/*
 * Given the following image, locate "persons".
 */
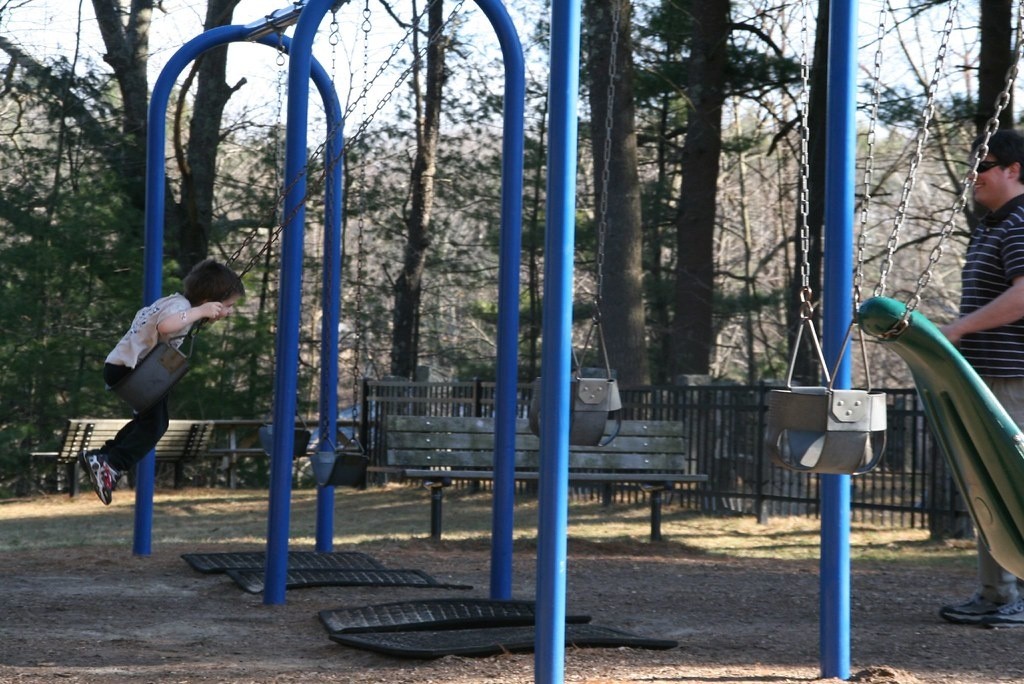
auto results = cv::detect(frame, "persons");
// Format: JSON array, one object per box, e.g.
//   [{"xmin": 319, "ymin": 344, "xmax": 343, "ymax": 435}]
[
  {"xmin": 936, "ymin": 130, "xmax": 1024, "ymax": 628},
  {"xmin": 78, "ymin": 259, "xmax": 245, "ymax": 506}
]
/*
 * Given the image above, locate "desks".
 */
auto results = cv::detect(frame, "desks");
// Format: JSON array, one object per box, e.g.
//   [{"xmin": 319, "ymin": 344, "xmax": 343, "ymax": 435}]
[{"xmin": 211, "ymin": 420, "xmax": 382, "ymax": 491}]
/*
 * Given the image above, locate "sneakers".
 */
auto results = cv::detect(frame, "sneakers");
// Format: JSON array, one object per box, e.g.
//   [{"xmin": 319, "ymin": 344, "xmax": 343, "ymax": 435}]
[
  {"xmin": 86, "ymin": 454, "xmax": 120, "ymax": 506},
  {"xmin": 939, "ymin": 595, "xmax": 1024, "ymax": 627},
  {"xmin": 79, "ymin": 450, "xmax": 92, "ymax": 479}
]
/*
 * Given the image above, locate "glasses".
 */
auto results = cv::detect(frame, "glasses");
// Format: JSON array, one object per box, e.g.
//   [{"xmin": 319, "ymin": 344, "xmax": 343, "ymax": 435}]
[{"xmin": 976, "ymin": 160, "xmax": 1000, "ymax": 174}]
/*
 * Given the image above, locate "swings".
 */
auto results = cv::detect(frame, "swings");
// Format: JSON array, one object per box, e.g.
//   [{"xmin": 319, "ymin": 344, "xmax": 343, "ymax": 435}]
[
  {"xmin": 514, "ymin": 1, "xmax": 626, "ymax": 452},
  {"xmin": 313, "ymin": 0, "xmax": 371, "ymax": 481},
  {"xmin": 133, "ymin": 0, "xmax": 466, "ymax": 402},
  {"xmin": 760, "ymin": 0, "xmax": 888, "ymax": 474},
  {"xmin": 259, "ymin": 23, "xmax": 312, "ymax": 459}
]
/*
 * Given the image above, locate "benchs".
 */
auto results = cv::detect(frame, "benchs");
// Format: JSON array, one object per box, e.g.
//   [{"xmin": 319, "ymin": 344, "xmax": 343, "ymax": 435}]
[
  {"xmin": 29, "ymin": 418, "xmax": 214, "ymax": 497},
  {"xmin": 200, "ymin": 447, "xmax": 361, "ymax": 455},
  {"xmin": 387, "ymin": 413, "xmax": 709, "ymax": 544}
]
